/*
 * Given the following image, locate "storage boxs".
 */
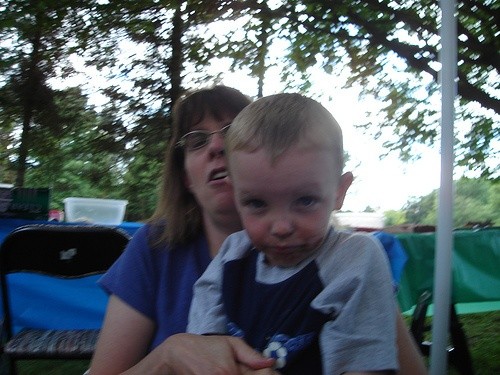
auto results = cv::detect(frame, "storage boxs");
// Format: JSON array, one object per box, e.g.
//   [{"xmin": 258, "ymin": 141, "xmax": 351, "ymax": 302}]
[{"xmin": 62, "ymin": 197, "xmax": 129, "ymax": 226}]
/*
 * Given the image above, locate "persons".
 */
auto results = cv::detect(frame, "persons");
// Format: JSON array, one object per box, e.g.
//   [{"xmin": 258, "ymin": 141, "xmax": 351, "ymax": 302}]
[
  {"xmin": 87, "ymin": 84, "xmax": 429, "ymax": 375},
  {"xmin": 185, "ymin": 92, "xmax": 400, "ymax": 375}
]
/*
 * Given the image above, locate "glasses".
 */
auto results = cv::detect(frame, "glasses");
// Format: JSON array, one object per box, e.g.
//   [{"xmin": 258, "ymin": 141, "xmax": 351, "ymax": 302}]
[{"xmin": 176, "ymin": 124, "xmax": 231, "ymax": 149}]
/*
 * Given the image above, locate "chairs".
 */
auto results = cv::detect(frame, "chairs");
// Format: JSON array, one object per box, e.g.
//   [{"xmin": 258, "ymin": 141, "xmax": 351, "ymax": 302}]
[{"xmin": 0, "ymin": 224, "xmax": 132, "ymax": 375}]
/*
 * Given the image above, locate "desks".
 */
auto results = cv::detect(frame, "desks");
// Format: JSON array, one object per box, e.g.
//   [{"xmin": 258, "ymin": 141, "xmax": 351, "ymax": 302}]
[{"xmin": 0, "ymin": 219, "xmax": 144, "ymax": 247}]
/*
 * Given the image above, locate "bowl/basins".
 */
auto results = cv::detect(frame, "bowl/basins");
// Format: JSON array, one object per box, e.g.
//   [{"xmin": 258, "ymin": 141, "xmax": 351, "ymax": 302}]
[
  {"xmin": 334, "ymin": 212, "xmax": 383, "ymax": 233},
  {"xmin": 62, "ymin": 197, "xmax": 128, "ymax": 225}
]
